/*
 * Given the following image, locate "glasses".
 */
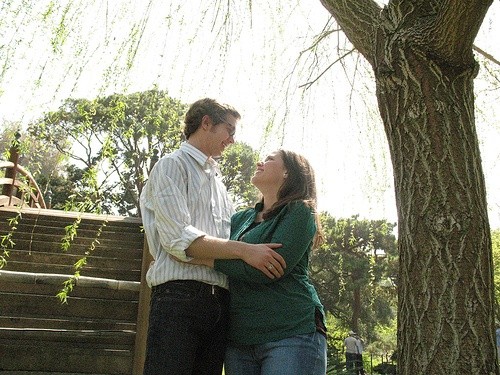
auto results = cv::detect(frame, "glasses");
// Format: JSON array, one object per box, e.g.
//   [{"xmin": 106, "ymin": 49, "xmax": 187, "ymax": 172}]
[{"xmin": 217, "ymin": 116, "xmax": 236, "ymax": 136}]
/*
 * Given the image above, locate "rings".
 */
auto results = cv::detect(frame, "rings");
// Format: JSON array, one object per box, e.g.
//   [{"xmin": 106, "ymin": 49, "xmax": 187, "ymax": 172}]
[{"xmin": 268, "ymin": 264, "xmax": 273, "ymax": 270}]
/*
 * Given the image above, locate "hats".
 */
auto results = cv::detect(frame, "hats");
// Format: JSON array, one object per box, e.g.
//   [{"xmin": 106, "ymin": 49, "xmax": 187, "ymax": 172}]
[{"xmin": 349, "ymin": 330, "xmax": 356, "ymax": 336}]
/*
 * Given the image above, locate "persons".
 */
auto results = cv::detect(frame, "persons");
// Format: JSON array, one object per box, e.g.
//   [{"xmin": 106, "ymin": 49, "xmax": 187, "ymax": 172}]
[
  {"xmin": 169, "ymin": 148, "xmax": 327, "ymax": 375},
  {"xmin": 343, "ymin": 331, "xmax": 359, "ymax": 373},
  {"xmin": 353, "ymin": 333, "xmax": 365, "ymax": 375},
  {"xmin": 139, "ymin": 98, "xmax": 287, "ymax": 375},
  {"xmin": 496, "ymin": 323, "xmax": 500, "ymax": 366}
]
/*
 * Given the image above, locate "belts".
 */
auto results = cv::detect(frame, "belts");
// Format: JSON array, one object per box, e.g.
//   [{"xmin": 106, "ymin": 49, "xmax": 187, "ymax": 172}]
[{"xmin": 151, "ymin": 279, "xmax": 231, "ymax": 302}]
